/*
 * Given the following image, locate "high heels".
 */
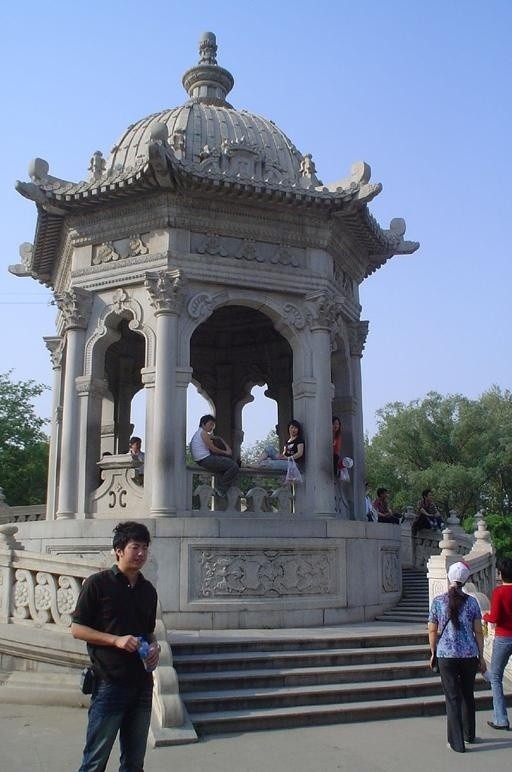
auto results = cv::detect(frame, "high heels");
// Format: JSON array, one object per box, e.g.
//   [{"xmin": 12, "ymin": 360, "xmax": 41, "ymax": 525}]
[{"xmin": 487, "ymin": 721, "xmax": 509, "ymax": 731}]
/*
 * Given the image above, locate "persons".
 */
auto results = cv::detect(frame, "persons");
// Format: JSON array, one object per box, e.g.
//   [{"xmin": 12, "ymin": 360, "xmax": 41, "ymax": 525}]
[
  {"xmin": 412, "ymin": 489, "xmax": 442, "ymax": 535},
  {"xmin": 427, "ymin": 560, "xmax": 489, "ymax": 752},
  {"xmin": 374, "ymin": 488, "xmax": 404, "ymax": 524},
  {"xmin": 239, "ymin": 420, "xmax": 304, "ymax": 472},
  {"xmin": 484, "ymin": 558, "xmax": 512, "ymax": 730},
  {"xmin": 71, "ymin": 520, "xmax": 163, "ymax": 772},
  {"xmin": 126, "ymin": 437, "xmax": 145, "ymax": 487},
  {"xmin": 331, "ymin": 416, "xmax": 341, "ymax": 477},
  {"xmin": 100, "ymin": 451, "xmax": 112, "ymax": 484},
  {"xmin": 190, "ymin": 415, "xmax": 238, "ymax": 496},
  {"xmin": 366, "ymin": 482, "xmax": 373, "ymax": 522}
]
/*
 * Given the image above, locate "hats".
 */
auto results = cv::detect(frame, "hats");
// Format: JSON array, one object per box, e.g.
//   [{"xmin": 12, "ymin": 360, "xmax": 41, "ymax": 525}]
[{"xmin": 447, "ymin": 562, "xmax": 470, "ymax": 584}]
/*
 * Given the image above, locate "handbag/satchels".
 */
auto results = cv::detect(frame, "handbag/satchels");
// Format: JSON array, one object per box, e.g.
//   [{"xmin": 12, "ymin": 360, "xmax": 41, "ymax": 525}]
[{"xmin": 80, "ymin": 666, "xmax": 96, "ymax": 694}]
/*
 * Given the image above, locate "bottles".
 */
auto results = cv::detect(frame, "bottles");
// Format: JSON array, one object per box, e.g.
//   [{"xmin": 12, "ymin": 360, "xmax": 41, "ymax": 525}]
[
  {"xmin": 481, "ymin": 619, "xmax": 488, "ymax": 639},
  {"xmin": 135, "ymin": 635, "xmax": 155, "ymax": 672},
  {"xmin": 478, "ymin": 664, "xmax": 492, "ymax": 682}
]
